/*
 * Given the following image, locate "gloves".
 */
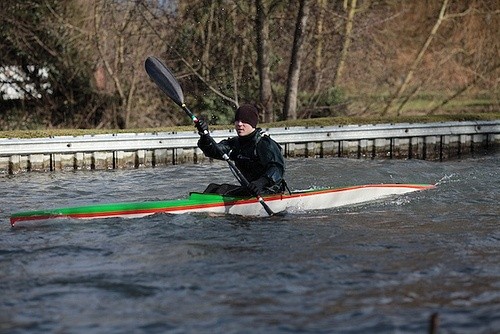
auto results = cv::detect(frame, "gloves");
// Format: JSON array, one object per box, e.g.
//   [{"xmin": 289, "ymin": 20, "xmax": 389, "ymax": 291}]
[
  {"xmin": 247, "ymin": 176, "xmax": 271, "ymax": 197},
  {"xmin": 194, "ymin": 120, "xmax": 209, "ymax": 136}
]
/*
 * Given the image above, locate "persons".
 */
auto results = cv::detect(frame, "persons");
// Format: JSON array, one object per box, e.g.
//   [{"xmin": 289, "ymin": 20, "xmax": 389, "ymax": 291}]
[{"xmin": 196, "ymin": 104, "xmax": 286, "ymax": 196}]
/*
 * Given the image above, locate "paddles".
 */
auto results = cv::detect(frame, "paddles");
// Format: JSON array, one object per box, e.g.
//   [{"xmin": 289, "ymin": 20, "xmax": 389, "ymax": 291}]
[{"xmin": 143, "ymin": 55, "xmax": 284, "ymax": 218}]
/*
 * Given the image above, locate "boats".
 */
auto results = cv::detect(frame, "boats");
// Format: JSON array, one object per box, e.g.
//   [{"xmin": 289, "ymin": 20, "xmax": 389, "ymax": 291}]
[{"xmin": 10, "ymin": 183, "xmax": 438, "ymax": 227}]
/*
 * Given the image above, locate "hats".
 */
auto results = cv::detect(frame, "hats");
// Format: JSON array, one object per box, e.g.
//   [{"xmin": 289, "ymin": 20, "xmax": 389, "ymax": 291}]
[{"xmin": 234, "ymin": 104, "xmax": 259, "ymax": 128}]
